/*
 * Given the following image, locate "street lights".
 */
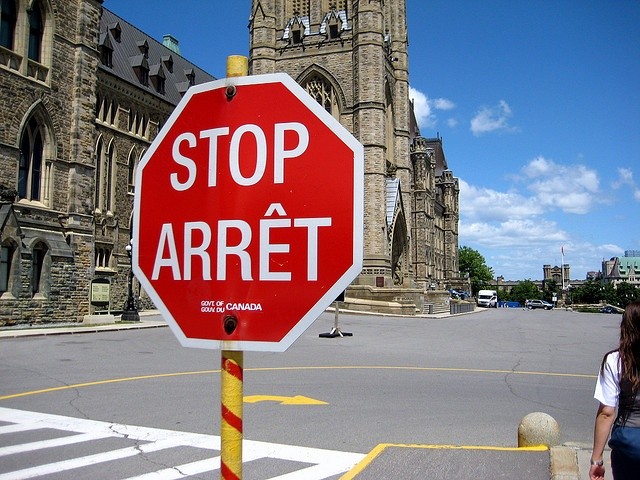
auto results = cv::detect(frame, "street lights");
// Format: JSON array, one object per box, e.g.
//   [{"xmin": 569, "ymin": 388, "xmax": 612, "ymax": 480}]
[
  {"xmin": 600, "ymin": 286, "xmax": 605, "ymax": 301},
  {"xmin": 496, "ymin": 274, "xmax": 505, "ymax": 303},
  {"xmin": 626, "ymin": 293, "xmax": 634, "ymax": 303}
]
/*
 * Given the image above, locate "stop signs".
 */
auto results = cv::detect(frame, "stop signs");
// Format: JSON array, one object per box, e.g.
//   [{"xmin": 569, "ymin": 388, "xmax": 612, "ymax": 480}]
[{"xmin": 130, "ymin": 72, "xmax": 363, "ymax": 349}]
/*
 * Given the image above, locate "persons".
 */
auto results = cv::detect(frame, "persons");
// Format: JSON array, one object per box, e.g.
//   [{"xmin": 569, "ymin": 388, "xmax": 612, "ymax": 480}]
[{"xmin": 588, "ymin": 304, "xmax": 640, "ymax": 480}]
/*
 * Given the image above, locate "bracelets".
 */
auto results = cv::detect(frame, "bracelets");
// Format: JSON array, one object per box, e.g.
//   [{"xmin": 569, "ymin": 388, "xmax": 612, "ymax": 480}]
[{"xmin": 590, "ymin": 460, "xmax": 605, "ymax": 466}]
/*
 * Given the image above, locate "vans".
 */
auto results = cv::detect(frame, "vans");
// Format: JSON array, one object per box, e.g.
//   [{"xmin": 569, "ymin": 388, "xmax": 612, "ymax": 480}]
[{"xmin": 478, "ymin": 290, "xmax": 497, "ymax": 308}]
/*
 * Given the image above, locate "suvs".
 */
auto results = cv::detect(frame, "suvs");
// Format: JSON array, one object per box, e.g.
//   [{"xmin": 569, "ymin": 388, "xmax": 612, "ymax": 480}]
[{"xmin": 526, "ymin": 299, "xmax": 554, "ymax": 310}]
